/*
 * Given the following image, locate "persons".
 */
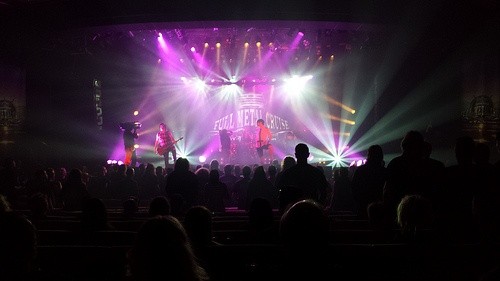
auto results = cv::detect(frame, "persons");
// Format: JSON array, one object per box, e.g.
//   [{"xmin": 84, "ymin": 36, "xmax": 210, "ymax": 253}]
[
  {"xmin": 310, "ymin": 130, "xmax": 500, "ymax": 251},
  {"xmin": 0, "ymin": 211, "xmax": 43, "ymax": 281},
  {"xmin": 257, "ymin": 200, "xmax": 357, "ymax": 281},
  {"xmin": 256, "ymin": 119, "xmax": 275, "ymax": 166},
  {"xmin": 219, "ymin": 130, "xmax": 233, "ymax": 166},
  {"xmin": 285, "ymin": 131, "xmax": 298, "ymax": 158},
  {"xmin": 123, "ymin": 124, "xmax": 139, "ymax": 170},
  {"xmin": 155, "ymin": 122, "xmax": 177, "ymax": 168},
  {"xmin": 0, "ymin": 143, "xmax": 311, "ymax": 244},
  {"xmin": 126, "ymin": 216, "xmax": 213, "ymax": 281}
]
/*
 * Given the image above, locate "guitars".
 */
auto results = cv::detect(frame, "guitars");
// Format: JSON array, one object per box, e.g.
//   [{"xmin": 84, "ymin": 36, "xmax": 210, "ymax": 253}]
[{"xmin": 157, "ymin": 137, "xmax": 183, "ymax": 155}]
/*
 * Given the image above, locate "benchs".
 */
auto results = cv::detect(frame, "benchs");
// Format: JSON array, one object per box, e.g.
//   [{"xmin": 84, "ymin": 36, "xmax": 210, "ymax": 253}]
[{"xmin": 36, "ymin": 209, "xmax": 402, "ymax": 281}]
